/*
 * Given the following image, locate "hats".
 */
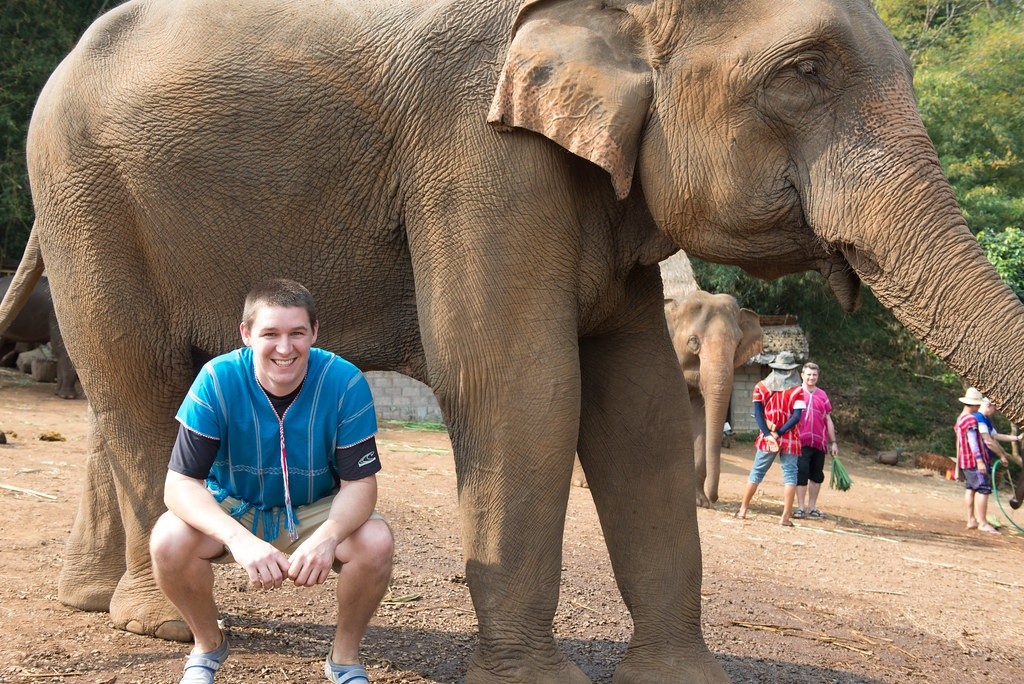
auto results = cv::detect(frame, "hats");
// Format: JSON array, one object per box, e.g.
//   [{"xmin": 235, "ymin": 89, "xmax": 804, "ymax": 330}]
[
  {"xmin": 765, "ymin": 351, "xmax": 800, "ymax": 391},
  {"xmin": 959, "ymin": 387, "xmax": 984, "ymax": 405}
]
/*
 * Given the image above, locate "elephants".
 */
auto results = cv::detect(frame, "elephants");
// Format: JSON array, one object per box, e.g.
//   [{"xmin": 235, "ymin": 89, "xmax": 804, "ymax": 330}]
[{"xmin": 1, "ymin": 0, "xmax": 1024, "ymax": 684}]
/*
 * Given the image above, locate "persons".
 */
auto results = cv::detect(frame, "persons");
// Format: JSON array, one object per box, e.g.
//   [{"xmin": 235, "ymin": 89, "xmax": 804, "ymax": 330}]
[
  {"xmin": 973, "ymin": 397, "xmax": 1023, "ymax": 529},
  {"xmin": 148, "ymin": 277, "xmax": 394, "ymax": 684},
  {"xmin": 953, "ymin": 387, "xmax": 1001, "ymax": 536},
  {"xmin": 736, "ymin": 351, "xmax": 807, "ymax": 527},
  {"xmin": 792, "ymin": 362, "xmax": 839, "ymax": 519}
]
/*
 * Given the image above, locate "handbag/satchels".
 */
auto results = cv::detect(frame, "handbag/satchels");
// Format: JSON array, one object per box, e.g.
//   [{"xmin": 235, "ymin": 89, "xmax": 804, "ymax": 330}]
[{"xmin": 954, "ymin": 454, "xmax": 965, "ymax": 482}]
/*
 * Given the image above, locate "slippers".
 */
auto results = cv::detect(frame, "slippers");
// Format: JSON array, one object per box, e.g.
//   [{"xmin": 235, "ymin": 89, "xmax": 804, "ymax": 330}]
[
  {"xmin": 809, "ymin": 510, "xmax": 824, "ymax": 518},
  {"xmin": 735, "ymin": 510, "xmax": 745, "ymax": 520},
  {"xmin": 792, "ymin": 509, "xmax": 806, "ymax": 519},
  {"xmin": 782, "ymin": 521, "xmax": 795, "ymax": 527}
]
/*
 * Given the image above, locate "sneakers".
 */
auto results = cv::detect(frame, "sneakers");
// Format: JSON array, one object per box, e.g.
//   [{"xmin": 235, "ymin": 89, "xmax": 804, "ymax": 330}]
[
  {"xmin": 323, "ymin": 645, "xmax": 369, "ymax": 684},
  {"xmin": 178, "ymin": 629, "xmax": 230, "ymax": 684}
]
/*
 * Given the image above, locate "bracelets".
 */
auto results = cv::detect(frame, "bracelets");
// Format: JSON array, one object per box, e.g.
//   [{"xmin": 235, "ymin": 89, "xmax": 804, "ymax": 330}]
[
  {"xmin": 831, "ymin": 441, "xmax": 836, "ymax": 444},
  {"xmin": 975, "ymin": 455, "xmax": 982, "ymax": 459},
  {"xmin": 1016, "ymin": 436, "xmax": 1019, "ymax": 442}
]
